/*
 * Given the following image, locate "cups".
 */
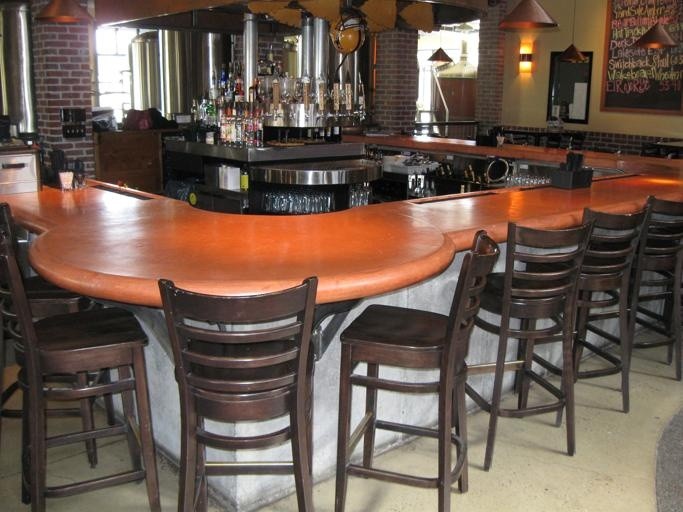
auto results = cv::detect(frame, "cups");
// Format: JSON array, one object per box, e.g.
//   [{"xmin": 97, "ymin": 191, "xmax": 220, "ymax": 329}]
[
  {"xmin": 58, "ymin": 171, "xmax": 73, "ymax": 190},
  {"xmin": 263, "ymin": 184, "xmax": 370, "ymax": 215}
]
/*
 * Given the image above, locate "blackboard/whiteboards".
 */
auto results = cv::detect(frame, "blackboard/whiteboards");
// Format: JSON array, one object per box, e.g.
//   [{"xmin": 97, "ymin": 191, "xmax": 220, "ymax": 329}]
[{"xmin": 600, "ymin": 0, "xmax": 683, "ymax": 117}]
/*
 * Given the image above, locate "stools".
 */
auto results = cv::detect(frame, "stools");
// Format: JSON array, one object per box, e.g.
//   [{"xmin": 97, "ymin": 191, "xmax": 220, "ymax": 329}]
[
  {"xmin": 1, "ymin": 203, "xmax": 162, "ymax": 512},
  {"xmin": 335, "ymin": 231, "xmax": 502, "ymax": 511},
  {"xmin": 158, "ymin": 276, "xmax": 316, "ymax": 512},
  {"xmin": 465, "ymin": 195, "xmax": 683, "ymax": 471}
]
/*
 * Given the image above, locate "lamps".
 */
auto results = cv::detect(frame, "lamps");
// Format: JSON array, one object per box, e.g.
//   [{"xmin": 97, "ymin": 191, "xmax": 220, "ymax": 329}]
[
  {"xmin": 425, "ymin": 30, "xmax": 454, "ymax": 63},
  {"xmin": 498, "ymin": 1, "xmax": 590, "ymax": 62},
  {"xmin": 633, "ymin": 1, "xmax": 679, "ymax": 50},
  {"xmin": 34, "ymin": 0, "xmax": 94, "ymax": 24}
]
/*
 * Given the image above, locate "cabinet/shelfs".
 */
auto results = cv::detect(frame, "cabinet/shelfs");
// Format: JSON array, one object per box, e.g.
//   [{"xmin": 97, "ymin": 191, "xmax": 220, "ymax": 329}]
[{"xmin": 93, "ymin": 125, "xmax": 190, "ymax": 195}]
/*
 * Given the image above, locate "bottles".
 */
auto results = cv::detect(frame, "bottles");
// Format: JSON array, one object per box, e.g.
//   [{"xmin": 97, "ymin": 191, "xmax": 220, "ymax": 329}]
[
  {"xmin": 506, "ymin": 173, "xmax": 552, "ymax": 188},
  {"xmin": 409, "ymin": 178, "xmax": 436, "ymax": 199},
  {"xmin": 190, "ymin": 60, "xmax": 341, "ymax": 149}
]
